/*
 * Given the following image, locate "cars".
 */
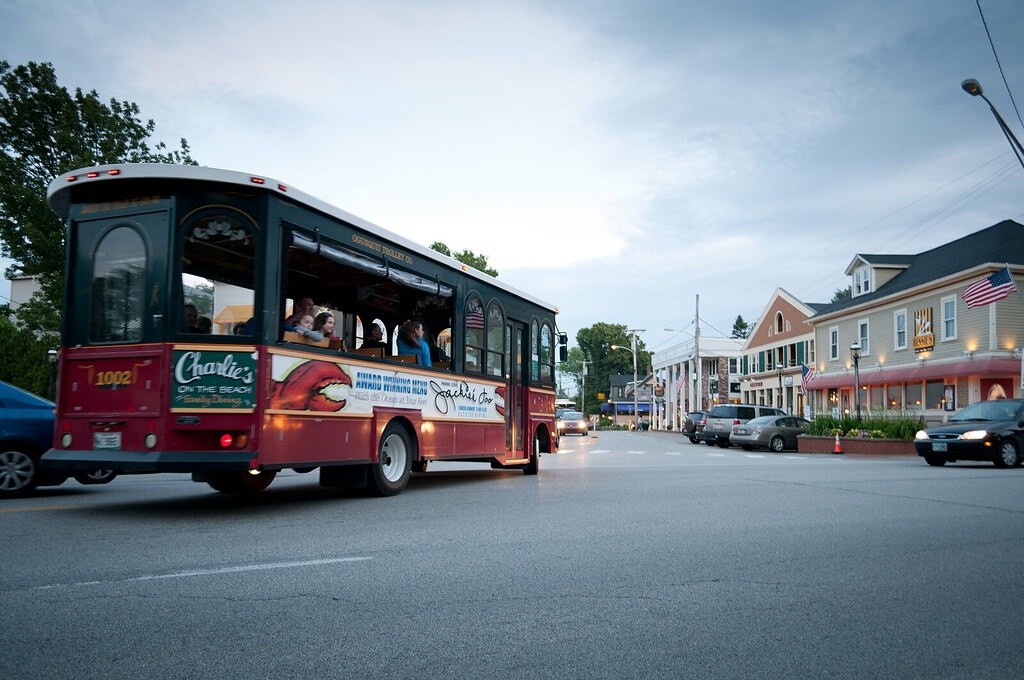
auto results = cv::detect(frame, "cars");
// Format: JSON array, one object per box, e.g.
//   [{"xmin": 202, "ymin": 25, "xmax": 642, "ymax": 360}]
[
  {"xmin": 729, "ymin": 415, "xmax": 816, "ymax": 453},
  {"xmin": 631, "ymin": 419, "xmax": 658, "ymax": 432},
  {"xmin": 556, "ymin": 399, "xmax": 588, "ymax": 436},
  {"xmin": 1, "ymin": 381, "xmax": 117, "ymax": 499},
  {"xmin": 913, "ymin": 399, "xmax": 1024, "ymax": 469}
]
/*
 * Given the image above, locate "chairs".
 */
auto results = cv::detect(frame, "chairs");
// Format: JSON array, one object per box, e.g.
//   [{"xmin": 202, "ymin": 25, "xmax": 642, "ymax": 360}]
[{"xmin": 284, "ymin": 331, "xmax": 493, "ymax": 376}]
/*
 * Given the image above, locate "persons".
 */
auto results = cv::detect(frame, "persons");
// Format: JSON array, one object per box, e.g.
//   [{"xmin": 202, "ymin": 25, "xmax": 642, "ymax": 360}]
[
  {"xmin": 360, "ymin": 323, "xmax": 390, "ymax": 356},
  {"xmin": 285, "ymin": 295, "xmax": 341, "ymax": 342},
  {"xmin": 396, "ymin": 320, "xmax": 432, "ymax": 368},
  {"xmin": 178, "ymin": 304, "xmax": 212, "ymax": 333},
  {"xmin": 423, "ymin": 333, "xmax": 446, "ymax": 362},
  {"xmin": 638, "ymin": 414, "xmax": 644, "ymax": 432}
]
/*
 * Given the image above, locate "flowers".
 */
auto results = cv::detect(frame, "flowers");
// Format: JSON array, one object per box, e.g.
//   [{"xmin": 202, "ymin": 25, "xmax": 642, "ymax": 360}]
[{"xmin": 830, "ymin": 429, "xmax": 886, "ymax": 438}]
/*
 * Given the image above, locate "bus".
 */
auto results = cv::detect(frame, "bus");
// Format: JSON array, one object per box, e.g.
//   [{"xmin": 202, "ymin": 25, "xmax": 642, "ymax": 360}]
[{"xmin": 40, "ymin": 163, "xmax": 568, "ymax": 498}]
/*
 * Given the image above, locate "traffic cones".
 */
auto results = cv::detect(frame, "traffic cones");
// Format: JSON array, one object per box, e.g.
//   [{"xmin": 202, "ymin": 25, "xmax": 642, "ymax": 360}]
[{"xmin": 831, "ymin": 432, "xmax": 844, "ymax": 454}]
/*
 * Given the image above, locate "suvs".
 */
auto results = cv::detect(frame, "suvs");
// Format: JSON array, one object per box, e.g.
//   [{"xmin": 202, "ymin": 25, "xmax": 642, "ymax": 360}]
[{"xmin": 682, "ymin": 404, "xmax": 788, "ymax": 448}]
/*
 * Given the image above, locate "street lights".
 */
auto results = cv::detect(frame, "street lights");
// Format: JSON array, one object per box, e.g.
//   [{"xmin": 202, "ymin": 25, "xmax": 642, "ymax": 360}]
[
  {"xmin": 664, "ymin": 327, "xmax": 699, "ymax": 413},
  {"xmin": 776, "ymin": 362, "xmax": 783, "ymax": 408},
  {"xmin": 611, "ymin": 345, "xmax": 638, "ymax": 432},
  {"xmin": 850, "ymin": 340, "xmax": 862, "ymax": 424}
]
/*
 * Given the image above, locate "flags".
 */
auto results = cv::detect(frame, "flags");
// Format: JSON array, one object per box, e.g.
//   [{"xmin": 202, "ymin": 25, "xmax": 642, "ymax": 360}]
[
  {"xmin": 801, "ymin": 364, "xmax": 814, "ymax": 395},
  {"xmin": 675, "ymin": 372, "xmax": 685, "ymax": 393},
  {"xmin": 961, "ymin": 267, "xmax": 1017, "ymax": 309}
]
